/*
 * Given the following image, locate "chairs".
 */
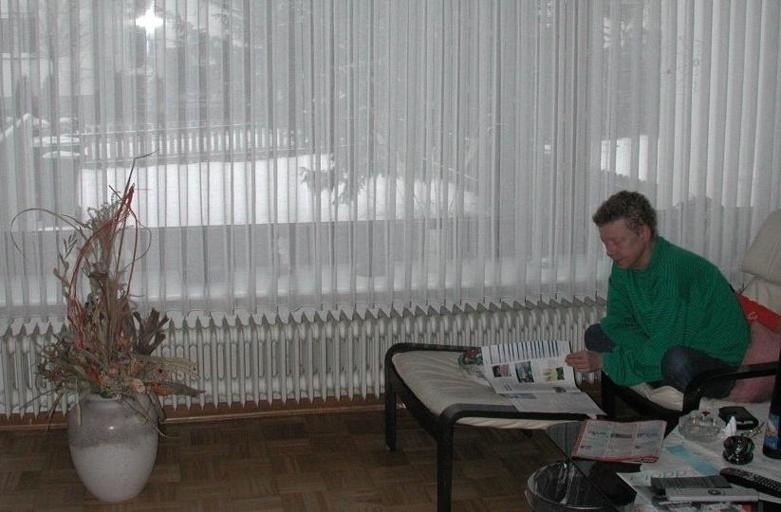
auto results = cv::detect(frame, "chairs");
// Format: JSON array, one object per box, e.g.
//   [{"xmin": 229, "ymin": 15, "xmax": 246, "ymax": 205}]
[{"xmin": 601, "ymin": 209, "xmax": 781, "ymax": 436}]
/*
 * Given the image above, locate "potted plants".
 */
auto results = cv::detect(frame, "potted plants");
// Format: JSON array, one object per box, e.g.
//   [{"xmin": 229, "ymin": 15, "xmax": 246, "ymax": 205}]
[{"xmin": 9, "ymin": 150, "xmax": 206, "ymax": 504}]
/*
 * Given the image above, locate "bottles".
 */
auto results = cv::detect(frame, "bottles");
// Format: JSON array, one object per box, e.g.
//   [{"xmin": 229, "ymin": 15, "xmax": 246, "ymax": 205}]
[{"xmin": 761, "ymin": 348, "xmax": 781, "ymax": 460}]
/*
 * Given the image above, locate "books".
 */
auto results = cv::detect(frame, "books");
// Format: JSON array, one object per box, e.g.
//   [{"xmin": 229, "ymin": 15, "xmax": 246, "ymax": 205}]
[
  {"xmin": 473, "ymin": 339, "xmax": 606, "ymax": 416},
  {"xmin": 572, "ymin": 418, "xmax": 672, "ymax": 464},
  {"xmin": 654, "ymin": 473, "xmax": 762, "ymax": 503}
]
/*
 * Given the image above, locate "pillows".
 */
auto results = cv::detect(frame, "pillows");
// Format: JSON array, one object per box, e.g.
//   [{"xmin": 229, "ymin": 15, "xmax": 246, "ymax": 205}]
[{"xmin": 724, "ymin": 321, "xmax": 781, "ymax": 403}]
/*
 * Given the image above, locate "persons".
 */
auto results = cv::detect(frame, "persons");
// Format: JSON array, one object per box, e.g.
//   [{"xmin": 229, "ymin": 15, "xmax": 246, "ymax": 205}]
[{"xmin": 564, "ymin": 188, "xmax": 749, "ymax": 397}]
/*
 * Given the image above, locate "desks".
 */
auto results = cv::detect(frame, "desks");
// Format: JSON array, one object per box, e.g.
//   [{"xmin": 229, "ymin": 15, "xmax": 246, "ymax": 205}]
[
  {"xmin": 545, "ymin": 412, "xmax": 781, "ymax": 512},
  {"xmin": 384, "ymin": 343, "xmax": 610, "ymax": 512}
]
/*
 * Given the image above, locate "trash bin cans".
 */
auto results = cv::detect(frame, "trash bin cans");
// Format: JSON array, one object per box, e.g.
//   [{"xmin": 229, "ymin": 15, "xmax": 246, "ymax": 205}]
[{"xmin": 525, "ymin": 462, "xmax": 606, "ymax": 511}]
